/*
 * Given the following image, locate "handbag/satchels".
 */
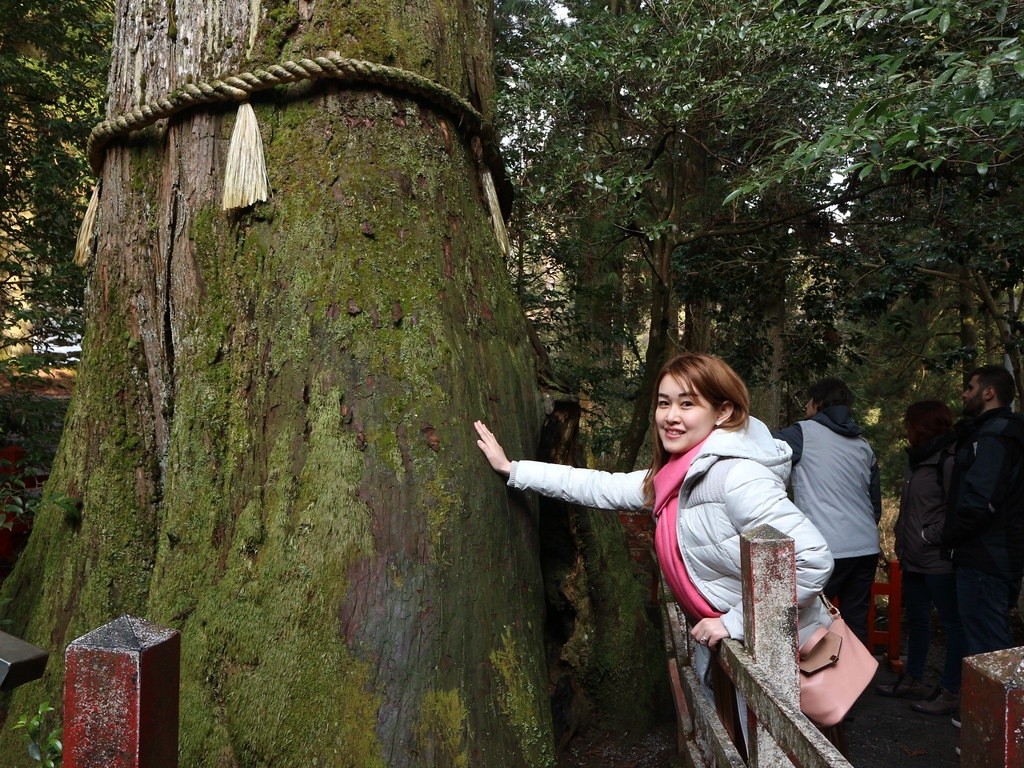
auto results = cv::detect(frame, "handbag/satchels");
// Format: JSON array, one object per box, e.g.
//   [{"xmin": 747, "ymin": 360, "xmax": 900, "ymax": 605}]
[{"xmin": 799, "ymin": 613, "xmax": 880, "ymax": 728}]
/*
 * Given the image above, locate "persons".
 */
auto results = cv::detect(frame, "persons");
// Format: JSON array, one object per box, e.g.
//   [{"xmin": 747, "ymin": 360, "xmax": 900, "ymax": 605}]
[
  {"xmin": 876, "ymin": 364, "xmax": 1024, "ymax": 755},
  {"xmin": 474, "ymin": 352, "xmax": 835, "ymax": 768},
  {"xmin": 773, "ymin": 376, "xmax": 882, "ymax": 644}
]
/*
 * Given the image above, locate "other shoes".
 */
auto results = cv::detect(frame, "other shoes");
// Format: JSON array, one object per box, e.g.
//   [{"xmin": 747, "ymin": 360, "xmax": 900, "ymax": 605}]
[{"xmin": 879, "ymin": 671, "xmax": 927, "ymax": 698}]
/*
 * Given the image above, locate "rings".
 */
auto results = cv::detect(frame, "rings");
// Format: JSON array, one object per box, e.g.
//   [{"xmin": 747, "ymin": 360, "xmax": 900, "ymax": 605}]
[{"xmin": 700, "ymin": 637, "xmax": 708, "ymax": 643}]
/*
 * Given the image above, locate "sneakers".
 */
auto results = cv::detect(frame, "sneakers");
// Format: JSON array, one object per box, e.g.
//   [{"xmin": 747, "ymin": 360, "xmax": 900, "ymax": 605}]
[{"xmin": 910, "ymin": 689, "xmax": 958, "ymax": 713}]
